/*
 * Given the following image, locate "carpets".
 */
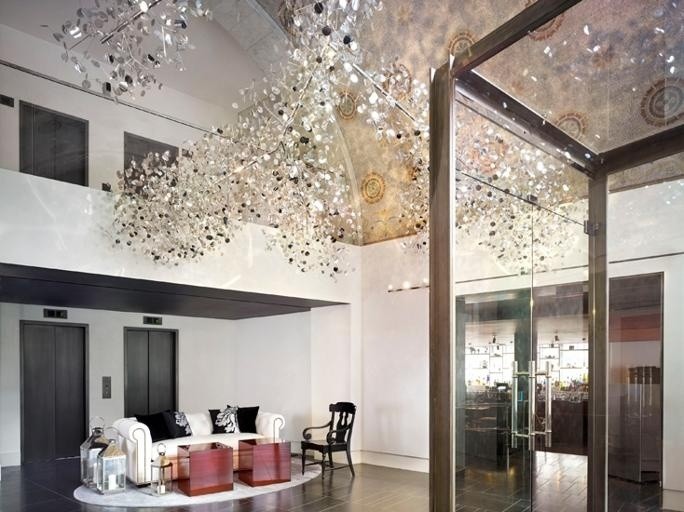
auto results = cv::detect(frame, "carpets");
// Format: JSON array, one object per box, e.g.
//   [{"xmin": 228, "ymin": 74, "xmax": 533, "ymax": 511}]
[{"xmin": 71, "ymin": 457, "xmax": 321, "ymax": 507}]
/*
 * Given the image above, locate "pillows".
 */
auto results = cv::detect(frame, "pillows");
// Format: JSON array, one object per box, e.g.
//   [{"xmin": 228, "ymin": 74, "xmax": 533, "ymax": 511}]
[{"xmin": 133, "ymin": 404, "xmax": 260, "ymax": 444}]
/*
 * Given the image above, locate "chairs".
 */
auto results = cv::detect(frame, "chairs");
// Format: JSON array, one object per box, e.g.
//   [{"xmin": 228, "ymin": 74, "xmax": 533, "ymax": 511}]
[{"xmin": 300, "ymin": 401, "xmax": 356, "ymax": 480}]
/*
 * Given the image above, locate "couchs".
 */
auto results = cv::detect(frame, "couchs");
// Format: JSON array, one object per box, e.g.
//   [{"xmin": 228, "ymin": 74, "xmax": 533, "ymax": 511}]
[{"xmin": 111, "ymin": 409, "xmax": 285, "ymax": 488}]
